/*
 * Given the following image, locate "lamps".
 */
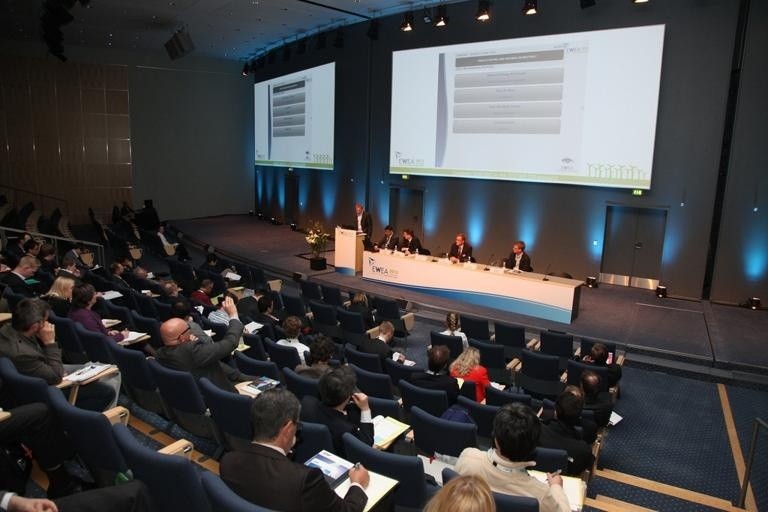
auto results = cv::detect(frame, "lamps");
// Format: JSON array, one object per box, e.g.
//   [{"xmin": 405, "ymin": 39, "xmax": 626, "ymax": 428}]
[
  {"xmin": 400, "ymin": 0, "xmax": 540, "ymax": 32},
  {"xmin": 241, "ymin": 28, "xmax": 344, "ymax": 75},
  {"xmin": 635, "ymin": 241, "xmax": 643, "ymax": 248}
]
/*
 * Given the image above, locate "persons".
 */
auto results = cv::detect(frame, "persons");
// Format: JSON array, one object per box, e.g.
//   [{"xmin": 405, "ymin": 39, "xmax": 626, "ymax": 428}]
[{"xmin": 1, "ymin": 201, "xmax": 622, "ymax": 511}]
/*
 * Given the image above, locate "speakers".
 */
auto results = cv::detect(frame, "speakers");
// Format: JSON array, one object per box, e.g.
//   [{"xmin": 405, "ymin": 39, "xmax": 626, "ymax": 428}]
[{"xmin": 164, "ymin": 28, "xmax": 196, "ymax": 61}]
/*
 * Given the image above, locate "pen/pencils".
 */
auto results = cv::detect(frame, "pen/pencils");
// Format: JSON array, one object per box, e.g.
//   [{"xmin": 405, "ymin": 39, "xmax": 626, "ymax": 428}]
[
  {"xmin": 354, "ymin": 462, "xmax": 360, "ymax": 472},
  {"xmin": 77, "ymin": 365, "xmax": 96, "ymax": 375},
  {"xmin": 545, "ymin": 468, "xmax": 562, "ymax": 482}
]
